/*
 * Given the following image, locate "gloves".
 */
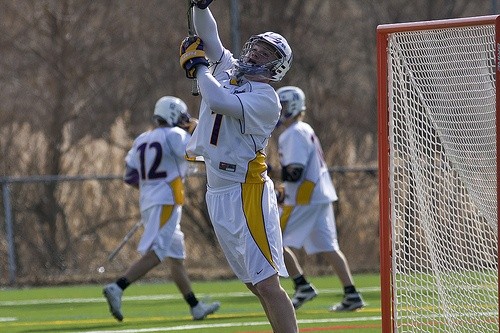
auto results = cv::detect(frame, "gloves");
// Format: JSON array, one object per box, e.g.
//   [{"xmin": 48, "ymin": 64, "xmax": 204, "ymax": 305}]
[
  {"xmin": 179, "ymin": 35, "xmax": 211, "ymax": 81},
  {"xmin": 192, "ymin": 0, "xmax": 214, "ymax": 12}
]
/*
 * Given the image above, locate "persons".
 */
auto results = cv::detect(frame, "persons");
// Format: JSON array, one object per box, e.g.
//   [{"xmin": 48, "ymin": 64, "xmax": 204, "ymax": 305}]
[
  {"xmin": 180, "ymin": 0, "xmax": 300, "ymax": 333},
  {"xmin": 275, "ymin": 86, "xmax": 367, "ymax": 312},
  {"xmin": 103, "ymin": 96, "xmax": 221, "ymax": 323}
]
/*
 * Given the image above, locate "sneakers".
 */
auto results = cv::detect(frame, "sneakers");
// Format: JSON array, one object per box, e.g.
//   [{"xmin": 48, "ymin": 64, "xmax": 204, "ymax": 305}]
[
  {"xmin": 102, "ymin": 283, "xmax": 125, "ymax": 321},
  {"xmin": 292, "ymin": 282, "xmax": 319, "ymax": 309},
  {"xmin": 329, "ymin": 292, "xmax": 366, "ymax": 314},
  {"xmin": 190, "ymin": 301, "xmax": 221, "ymax": 321}
]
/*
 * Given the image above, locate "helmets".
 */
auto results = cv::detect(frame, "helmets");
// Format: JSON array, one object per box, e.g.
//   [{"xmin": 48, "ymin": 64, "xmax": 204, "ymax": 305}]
[
  {"xmin": 153, "ymin": 95, "xmax": 192, "ymax": 128},
  {"xmin": 235, "ymin": 31, "xmax": 295, "ymax": 82},
  {"xmin": 274, "ymin": 86, "xmax": 307, "ymax": 126}
]
